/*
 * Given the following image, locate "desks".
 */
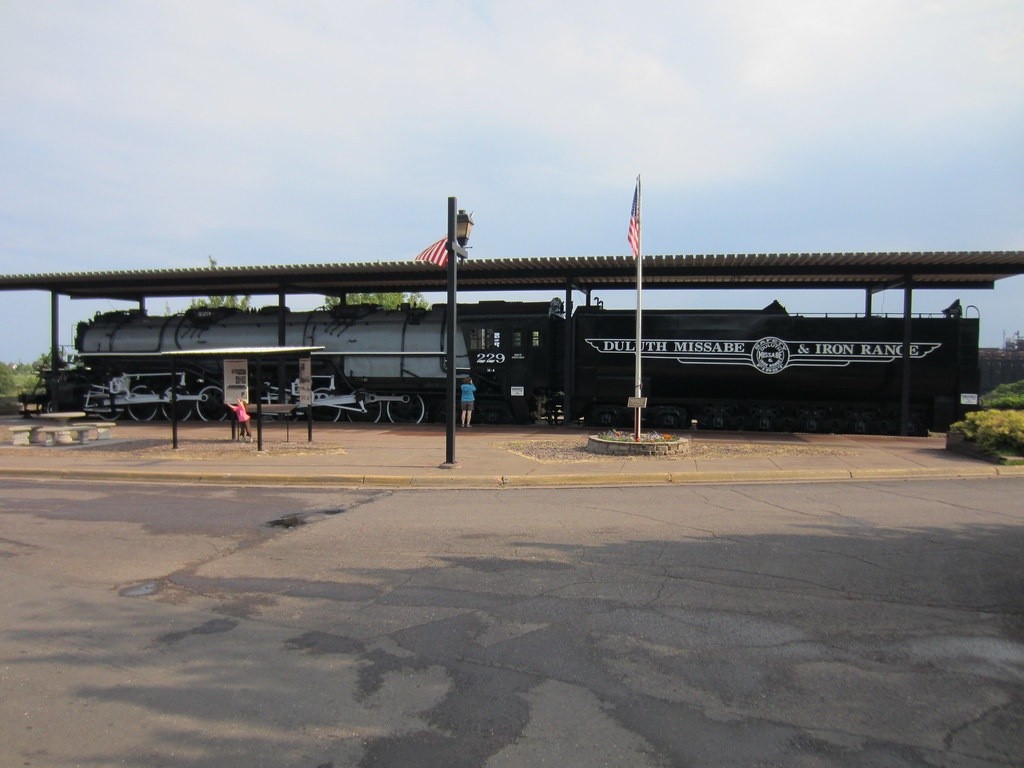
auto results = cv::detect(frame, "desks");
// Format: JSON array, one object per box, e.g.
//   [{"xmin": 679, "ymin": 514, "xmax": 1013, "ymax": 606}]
[{"xmin": 40, "ymin": 412, "xmax": 86, "ymax": 443}]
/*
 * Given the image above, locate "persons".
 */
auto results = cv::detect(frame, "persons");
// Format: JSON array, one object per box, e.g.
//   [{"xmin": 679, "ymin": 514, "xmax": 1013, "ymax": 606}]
[
  {"xmin": 226, "ymin": 399, "xmax": 253, "ymax": 443},
  {"xmin": 460, "ymin": 377, "xmax": 476, "ymax": 428}
]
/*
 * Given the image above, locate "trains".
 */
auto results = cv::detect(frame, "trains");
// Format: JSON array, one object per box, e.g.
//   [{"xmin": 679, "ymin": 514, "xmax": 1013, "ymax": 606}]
[{"xmin": 73, "ymin": 296, "xmax": 982, "ymax": 437}]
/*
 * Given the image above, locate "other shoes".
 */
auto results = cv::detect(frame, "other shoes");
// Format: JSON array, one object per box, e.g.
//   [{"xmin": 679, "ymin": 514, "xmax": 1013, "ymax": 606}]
[
  {"xmin": 467, "ymin": 424, "xmax": 472, "ymax": 428},
  {"xmin": 462, "ymin": 424, "xmax": 466, "ymax": 428}
]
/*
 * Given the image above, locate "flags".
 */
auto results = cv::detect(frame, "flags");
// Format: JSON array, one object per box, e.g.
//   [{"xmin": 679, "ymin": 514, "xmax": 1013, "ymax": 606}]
[
  {"xmin": 628, "ymin": 176, "xmax": 642, "ymax": 260},
  {"xmin": 415, "ymin": 234, "xmax": 448, "ymax": 267}
]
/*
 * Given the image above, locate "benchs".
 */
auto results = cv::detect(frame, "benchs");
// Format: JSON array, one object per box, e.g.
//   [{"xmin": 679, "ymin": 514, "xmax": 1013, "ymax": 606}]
[
  {"xmin": 37, "ymin": 426, "xmax": 97, "ymax": 446},
  {"xmin": 72, "ymin": 422, "xmax": 116, "ymax": 439},
  {"xmin": 9, "ymin": 425, "xmax": 39, "ymax": 445}
]
